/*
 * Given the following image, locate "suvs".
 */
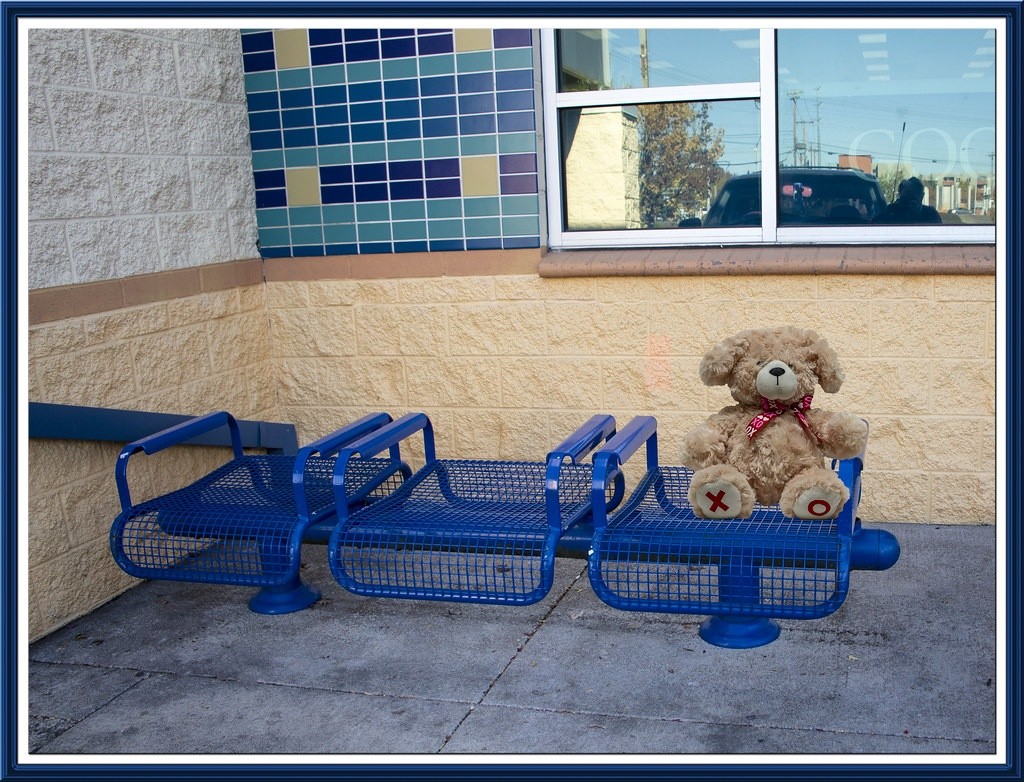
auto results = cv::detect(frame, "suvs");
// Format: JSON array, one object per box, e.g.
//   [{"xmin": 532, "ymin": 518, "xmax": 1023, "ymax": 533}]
[{"xmin": 677, "ymin": 164, "xmax": 890, "ymax": 228}]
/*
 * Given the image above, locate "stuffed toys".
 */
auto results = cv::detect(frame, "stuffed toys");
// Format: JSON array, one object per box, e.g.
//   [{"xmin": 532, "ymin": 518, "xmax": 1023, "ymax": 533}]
[{"xmin": 680, "ymin": 325, "xmax": 868, "ymax": 520}]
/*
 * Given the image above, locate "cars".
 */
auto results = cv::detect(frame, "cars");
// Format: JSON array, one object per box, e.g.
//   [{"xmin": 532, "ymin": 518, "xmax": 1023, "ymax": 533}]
[{"xmin": 948, "ymin": 207, "xmax": 973, "ymax": 215}]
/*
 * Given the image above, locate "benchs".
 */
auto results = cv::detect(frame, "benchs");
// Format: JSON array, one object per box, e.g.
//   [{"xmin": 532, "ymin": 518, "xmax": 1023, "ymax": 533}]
[{"xmin": 109, "ymin": 412, "xmax": 898, "ymax": 652}]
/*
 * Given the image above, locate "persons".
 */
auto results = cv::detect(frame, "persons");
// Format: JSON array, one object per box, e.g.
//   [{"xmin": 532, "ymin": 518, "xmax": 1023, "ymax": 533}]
[{"xmin": 871, "ymin": 176, "xmax": 942, "ymax": 226}]
